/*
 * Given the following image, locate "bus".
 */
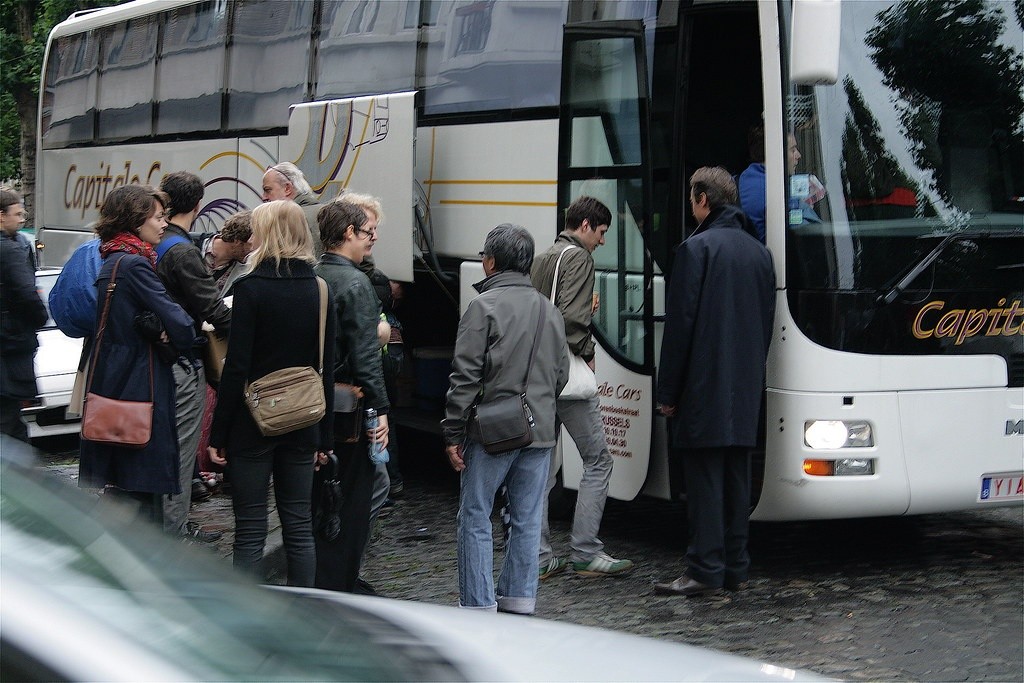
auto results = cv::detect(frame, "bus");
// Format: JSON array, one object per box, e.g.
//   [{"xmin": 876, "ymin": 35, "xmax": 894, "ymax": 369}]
[{"xmin": 31, "ymin": 1, "xmax": 1023, "ymax": 534}]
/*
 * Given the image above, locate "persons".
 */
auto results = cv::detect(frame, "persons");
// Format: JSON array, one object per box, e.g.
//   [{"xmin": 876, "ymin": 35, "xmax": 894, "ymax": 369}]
[
  {"xmin": 0, "ymin": 161, "xmax": 402, "ymax": 595},
  {"xmin": 436, "ymin": 223, "xmax": 570, "ymax": 617},
  {"xmin": 652, "ymin": 167, "xmax": 777, "ymax": 596},
  {"xmin": 526, "ymin": 195, "xmax": 633, "ymax": 580},
  {"xmin": 736, "ymin": 121, "xmax": 825, "ymax": 245}
]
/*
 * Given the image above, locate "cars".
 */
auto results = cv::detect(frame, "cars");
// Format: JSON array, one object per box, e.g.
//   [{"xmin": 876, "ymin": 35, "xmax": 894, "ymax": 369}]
[
  {"xmin": 1, "ymin": 436, "xmax": 860, "ymax": 683},
  {"xmin": 21, "ymin": 268, "xmax": 84, "ymax": 442}
]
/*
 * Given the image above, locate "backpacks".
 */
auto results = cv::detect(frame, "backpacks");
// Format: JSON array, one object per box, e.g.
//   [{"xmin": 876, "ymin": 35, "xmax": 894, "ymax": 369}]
[{"xmin": 48, "ymin": 235, "xmax": 192, "ymax": 338}]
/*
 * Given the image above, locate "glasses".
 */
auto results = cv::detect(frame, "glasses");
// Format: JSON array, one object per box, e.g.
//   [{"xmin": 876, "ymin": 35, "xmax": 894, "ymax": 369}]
[
  {"xmin": 359, "ymin": 227, "xmax": 374, "ymax": 241},
  {"xmin": 266, "ymin": 164, "xmax": 294, "ymax": 186},
  {"xmin": 477, "ymin": 251, "xmax": 490, "ymax": 261}
]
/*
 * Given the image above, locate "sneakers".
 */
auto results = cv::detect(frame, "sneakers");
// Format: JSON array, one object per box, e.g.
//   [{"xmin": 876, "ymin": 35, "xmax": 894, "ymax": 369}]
[
  {"xmin": 538, "ymin": 557, "xmax": 568, "ymax": 580},
  {"xmin": 573, "ymin": 550, "xmax": 633, "ymax": 576},
  {"xmin": 186, "ymin": 521, "xmax": 222, "ymax": 542},
  {"xmin": 177, "ymin": 533, "xmax": 219, "ymax": 554}
]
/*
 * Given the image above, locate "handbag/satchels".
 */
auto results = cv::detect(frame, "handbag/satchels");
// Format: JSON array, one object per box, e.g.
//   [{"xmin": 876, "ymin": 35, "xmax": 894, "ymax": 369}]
[
  {"xmin": 80, "ymin": 393, "xmax": 153, "ymax": 445},
  {"xmin": 550, "ymin": 340, "xmax": 599, "ymax": 400},
  {"xmin": 322, "ymin": 382, "xmax": 364, "ymax": 443},
  {"xmin": 244, "ymin": 365, "xmax": 328, "ymax": 436},
  {"xmin": 473, "ymin": 393, "xmax": 535, "ymax": 455}
]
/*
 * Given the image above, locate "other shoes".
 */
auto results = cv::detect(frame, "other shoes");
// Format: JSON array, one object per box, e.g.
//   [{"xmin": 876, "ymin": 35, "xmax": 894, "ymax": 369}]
[
  {"xmin": 653, "ymin": 575, "xmax": 722, "ymax": 595},
  {"xmin": 191, "ymin": 478, "xmax": 210, "ymax": 500},
  {"xmin": 196, "ymin": 475, "xmax": 220, "ymax": 493},
  {"xmin": 726, "ymin": 578, "xmax": 750, "ymax": 593},
  {"xmin": 353, "ymin": 577, "xmax": 376, "ymax": 596}
]
